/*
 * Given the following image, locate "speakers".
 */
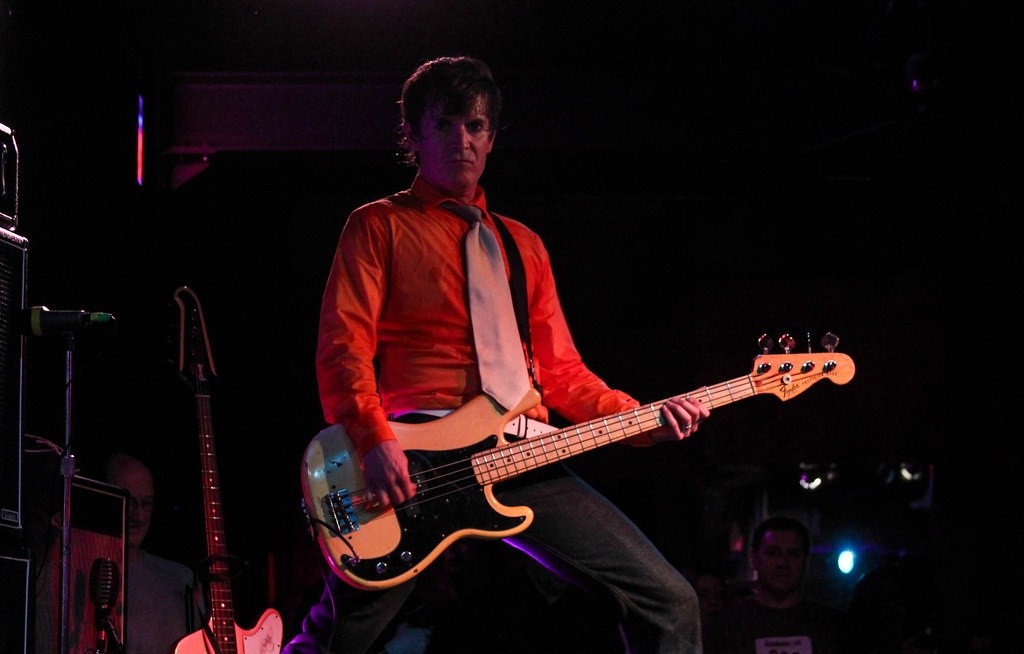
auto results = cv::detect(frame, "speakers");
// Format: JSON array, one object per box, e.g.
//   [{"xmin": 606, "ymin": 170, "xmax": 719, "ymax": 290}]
[
  {"xmin": 31, "ymin": 472, "xmax": 130, "ymax": 654},
  {"xmin": 1, "ymin": 227, "xmax": 30, "ymax": 550},
  {"xmin": 0, "ymin": 548, "xmax": 33, "ymax": 654}
]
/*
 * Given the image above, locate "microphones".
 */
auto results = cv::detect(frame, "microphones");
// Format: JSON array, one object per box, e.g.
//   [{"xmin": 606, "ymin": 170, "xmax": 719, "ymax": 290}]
[
  {"xmin": 90, "ymin": 558, "xmax": 119, "ymax": 654},
  {"xmin": 15, "ymin": 305, "xmax": 114, "ymax": 337}
]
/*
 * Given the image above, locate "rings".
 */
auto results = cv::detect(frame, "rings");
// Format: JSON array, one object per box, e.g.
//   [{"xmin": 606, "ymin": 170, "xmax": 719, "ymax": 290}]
[{"xmin": 684, "ymin": 425, "xmax": 691, "ymax": 429}]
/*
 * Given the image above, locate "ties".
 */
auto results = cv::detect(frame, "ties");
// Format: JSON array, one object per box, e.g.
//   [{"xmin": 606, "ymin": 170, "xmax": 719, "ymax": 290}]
[{"xmin": 441, "ymin": 201, "xmax": 531, "ymax": 411}]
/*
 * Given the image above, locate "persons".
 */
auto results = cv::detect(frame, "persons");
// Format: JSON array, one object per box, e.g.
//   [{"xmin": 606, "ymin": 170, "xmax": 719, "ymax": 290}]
[
  {"xmin": 682, "ymin": 518, "xmax": 870, "ymax": 654},
  {"xmin": 279, "ymin": 55, "xmax": 710, "ymax": 654},
  {"xmin": 34, "ymin": 453, "xmax": 358, "ymax": 654}
]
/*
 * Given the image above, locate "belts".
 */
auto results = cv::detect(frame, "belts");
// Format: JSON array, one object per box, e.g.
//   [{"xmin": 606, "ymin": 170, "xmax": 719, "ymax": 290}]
[{"xmin": 388, "ymin": 407, "xmax": 560, "ymax": 441}]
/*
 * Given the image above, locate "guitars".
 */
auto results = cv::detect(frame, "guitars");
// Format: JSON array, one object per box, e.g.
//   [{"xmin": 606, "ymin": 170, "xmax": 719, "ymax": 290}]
[
  {"xmin": 296, "ymin": 328, "xmax": 861, "ymax": 598},
  {"xmin": 168, "ymin": 281, "xmax": 287, "ymax": 654}
]
